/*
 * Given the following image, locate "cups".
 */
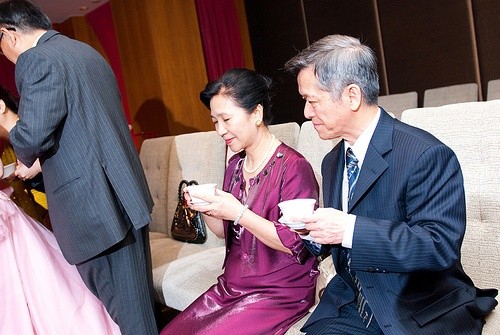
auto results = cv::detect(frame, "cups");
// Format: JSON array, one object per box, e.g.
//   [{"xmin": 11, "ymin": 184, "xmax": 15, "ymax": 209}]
[
  {"xmin": 186, "ymin": 183, "xmax": 216, "ymax": 202},
  {"xmin": 2, "ymin": 163, "xmax": 16, "ymax": 179},
  {"xmin": 277, "ymin": 198, "xmax": 317, "ymax": 221}
]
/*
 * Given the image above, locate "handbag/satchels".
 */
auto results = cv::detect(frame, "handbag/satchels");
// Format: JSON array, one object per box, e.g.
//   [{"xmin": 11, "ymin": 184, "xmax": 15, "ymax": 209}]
[{"xmin": 171, "ymin": 180, "xmax": 208, "ymax": 244}]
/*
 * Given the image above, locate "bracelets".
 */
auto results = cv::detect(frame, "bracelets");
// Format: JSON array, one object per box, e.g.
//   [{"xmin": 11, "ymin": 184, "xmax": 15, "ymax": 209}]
[{"xmin": 233, "ymin": 204, "xmax": 248, "ymax": 225}]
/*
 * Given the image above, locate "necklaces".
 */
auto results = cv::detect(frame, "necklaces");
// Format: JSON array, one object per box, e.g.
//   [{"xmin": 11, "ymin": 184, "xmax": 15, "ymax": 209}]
[{"xmin": 244, "ymin": 134, "xmax": 275, "ymax": 173}]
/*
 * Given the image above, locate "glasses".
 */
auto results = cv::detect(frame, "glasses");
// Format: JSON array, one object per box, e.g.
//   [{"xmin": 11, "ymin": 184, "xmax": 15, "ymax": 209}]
[{"xmin": 0, "ymin": 27, "xmax": 16, "ymax": 55}]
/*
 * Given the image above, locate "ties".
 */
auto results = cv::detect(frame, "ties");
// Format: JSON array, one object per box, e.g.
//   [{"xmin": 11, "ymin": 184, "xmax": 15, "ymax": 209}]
[{"xmin": 346, "ymin": 147, "xmax": 374, "ymax": 328}]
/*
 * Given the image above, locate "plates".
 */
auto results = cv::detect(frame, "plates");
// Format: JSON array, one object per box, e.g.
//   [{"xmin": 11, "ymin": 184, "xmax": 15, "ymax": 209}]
[{"xmin": 278, "ymin": 212, "xmax": 317, "ymax": 230}]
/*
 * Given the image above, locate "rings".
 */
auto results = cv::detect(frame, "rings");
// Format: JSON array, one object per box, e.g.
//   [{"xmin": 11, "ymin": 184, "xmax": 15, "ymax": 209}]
[{"xmin": 207, "ymin": 212, "xmax": 210, "ymax": 216}]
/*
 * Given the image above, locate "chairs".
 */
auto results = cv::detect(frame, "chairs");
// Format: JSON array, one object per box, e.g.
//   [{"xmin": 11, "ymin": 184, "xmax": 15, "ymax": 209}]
[{"xmin": 139, "ymin": 80, "xmax": 500, "ymax": 335}]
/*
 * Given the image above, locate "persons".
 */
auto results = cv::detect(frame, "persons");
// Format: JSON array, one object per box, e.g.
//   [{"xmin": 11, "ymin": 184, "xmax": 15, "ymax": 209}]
[
  {"xmin": 290, "ymin": 35, "xmax": 483, "ymax": 335},
  {"xmin": 0, "ymin": 89, "xmax": 122, "ymax": 335},
  {"xmin": 159, "ymin": 68, "xmax": 319, "ymax": 335},
  {"xmin": 0, "ymin": 0, "xmax": 159, "ymax": 335}
]
